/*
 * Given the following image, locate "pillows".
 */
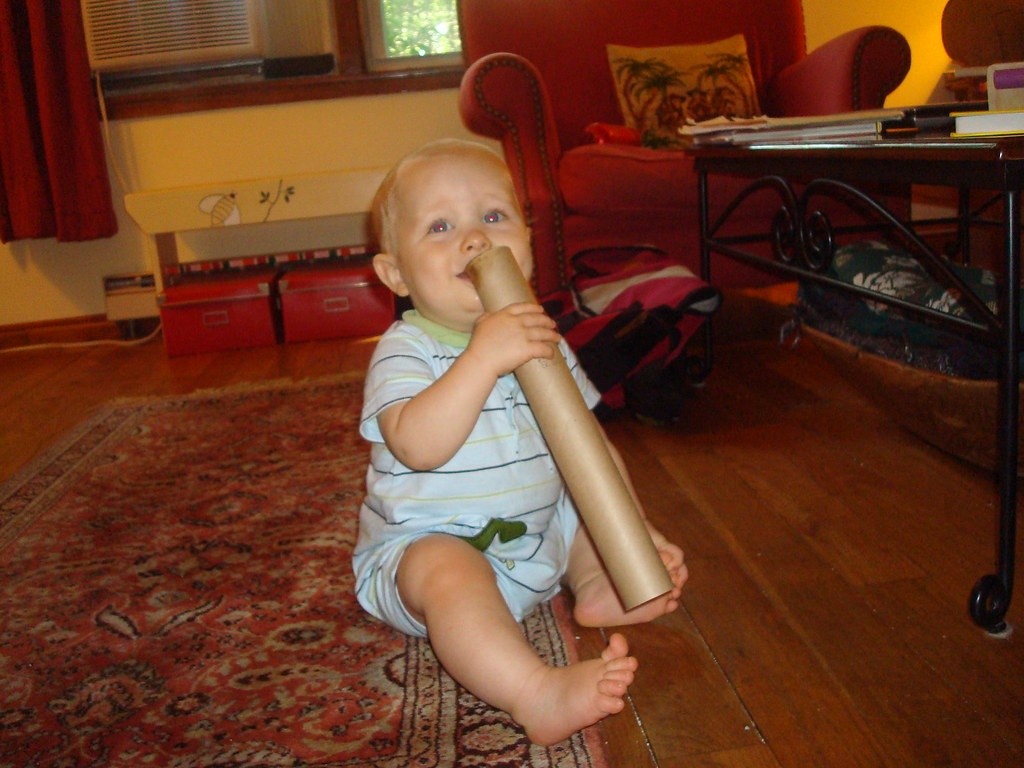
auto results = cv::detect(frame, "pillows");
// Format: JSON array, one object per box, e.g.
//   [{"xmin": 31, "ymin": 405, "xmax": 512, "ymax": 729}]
[{"xmin": 607, "ymin": 33, "xmax": 762, "ymax": 151}]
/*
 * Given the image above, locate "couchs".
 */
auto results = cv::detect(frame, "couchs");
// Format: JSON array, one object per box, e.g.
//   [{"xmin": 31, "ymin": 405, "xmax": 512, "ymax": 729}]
[{"xmin": 456, "ymin": 0, "xmax": 911, "ymax": 296}]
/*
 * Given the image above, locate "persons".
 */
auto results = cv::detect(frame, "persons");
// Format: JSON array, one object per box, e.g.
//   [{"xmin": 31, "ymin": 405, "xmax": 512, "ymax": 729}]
[{"xmin": 353, "ymin": 139, "xmax": 688, "ymax": 745}]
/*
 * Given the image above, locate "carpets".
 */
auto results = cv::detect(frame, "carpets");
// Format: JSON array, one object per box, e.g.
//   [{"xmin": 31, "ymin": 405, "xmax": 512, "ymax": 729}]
[{"xmin": 0, "ymin": 368, "xmax": 606, "ymax": 768}]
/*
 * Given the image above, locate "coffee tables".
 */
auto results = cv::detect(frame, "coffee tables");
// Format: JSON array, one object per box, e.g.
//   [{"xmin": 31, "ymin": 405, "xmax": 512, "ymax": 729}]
[{"xmin": 684, "ymin": 139, "xmax": 1024, "ymax": 632}]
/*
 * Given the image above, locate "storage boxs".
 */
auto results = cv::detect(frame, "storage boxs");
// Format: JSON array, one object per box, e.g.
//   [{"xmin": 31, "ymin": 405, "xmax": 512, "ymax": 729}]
[
  {"xmin": 281, "ymin": 257, "xmax": 395, "ymax": 342},
  {"xmin": 156, "ymin": 268, "xmax": 281, "ymax": 358}
]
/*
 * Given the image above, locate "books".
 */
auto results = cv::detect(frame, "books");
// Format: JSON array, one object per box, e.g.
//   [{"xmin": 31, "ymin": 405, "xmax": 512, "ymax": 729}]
[
  {"xmin": 949, "ymin": 110, "xmax": 1024, "ymax": 139},
  {"xmin": 678, "ymin": 110, "xmax": 904, "ymax": 146}
]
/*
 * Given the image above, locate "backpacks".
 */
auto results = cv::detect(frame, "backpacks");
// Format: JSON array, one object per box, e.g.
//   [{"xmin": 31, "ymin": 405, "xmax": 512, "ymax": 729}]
[{"xmin": 539, "ymin": 234, "xmax": 715, "ymax": 424}]
